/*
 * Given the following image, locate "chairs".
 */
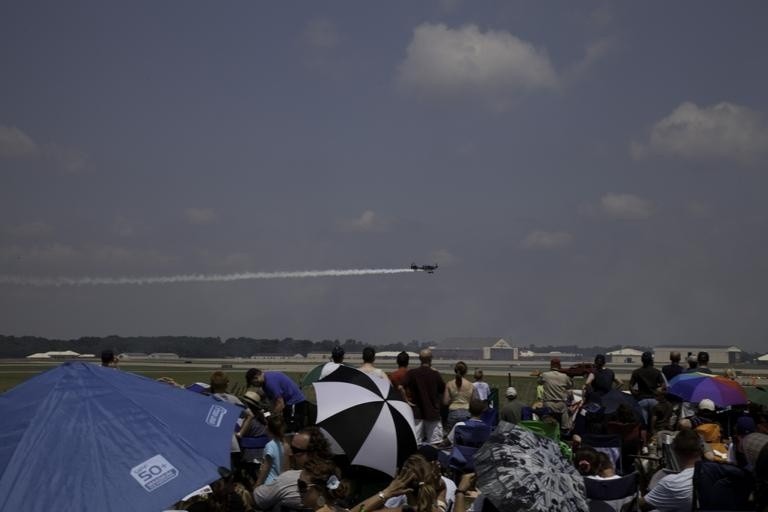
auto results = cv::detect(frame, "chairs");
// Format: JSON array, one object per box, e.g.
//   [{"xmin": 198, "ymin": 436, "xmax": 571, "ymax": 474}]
[
  {"xmin": 691, "ymin": 459, "xmax": 752, "ymax": 512},
  {"xmin": 241, "ymin": 436, "xmax": 268, "ymax": 462},
  {"xmin": 583, "ymin": 470, "xmax": 642, "ymax": 512},
  {"xmin": 452, "ymin": 424, "xmax": 491, "ymax": 487},
  {"xmin": 280, "ymin": 503, "xmax": 315, "ymax": 512}
]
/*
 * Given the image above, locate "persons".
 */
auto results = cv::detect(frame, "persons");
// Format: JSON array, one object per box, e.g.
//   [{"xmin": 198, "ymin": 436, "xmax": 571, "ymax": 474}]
[{"xmin": 180, "ymin": 346, "xmax": 767, "ymax": 512}]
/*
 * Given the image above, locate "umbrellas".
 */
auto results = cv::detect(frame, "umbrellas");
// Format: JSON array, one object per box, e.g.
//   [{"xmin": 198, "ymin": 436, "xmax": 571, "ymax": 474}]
[{"xmin": 2, "ymin": 358, "xmax": 244, "ymax": 512}]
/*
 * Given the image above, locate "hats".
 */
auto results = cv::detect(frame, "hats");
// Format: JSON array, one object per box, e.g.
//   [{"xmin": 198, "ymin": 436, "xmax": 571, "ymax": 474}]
[
  {"xmin": 505, "ymin": 386, "xmax": 517, "ymax": 399},
  {"xmin": 419, "ymin": 348, "xmax": 434, "ymax": 359},
  {"xmin": 734, "ymin": 416, "xmax": 756, "ymax": 434},
  {"xmin": 697, "ymin": 352, "xmax": 709, "ymax": 362},
  {"xmin": 239, "ymin": 390, "xmax": 261, "ymax": 409},
  {"xmin": 244, "ymin": 368, "xmax": 258, "ymax": 389},
  {"xmin": 551, "ymin": 358, "xmax": 561, "ymax": 366},
  {"xmin": 699, "ymin": 399, "xmax": 716, "ymax": 411}
]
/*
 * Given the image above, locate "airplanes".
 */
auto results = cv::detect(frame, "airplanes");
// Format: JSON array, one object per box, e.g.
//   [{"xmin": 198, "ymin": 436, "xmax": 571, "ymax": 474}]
[{"xmin": 408, "ymin": 259, "xmax": 441, "ymax": 276}]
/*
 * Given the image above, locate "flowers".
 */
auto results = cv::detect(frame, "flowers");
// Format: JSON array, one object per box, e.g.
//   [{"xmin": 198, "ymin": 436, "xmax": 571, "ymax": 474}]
[{"xmin": 326, "ymin": 475, "xmax": 340, "ymax": 489}]
